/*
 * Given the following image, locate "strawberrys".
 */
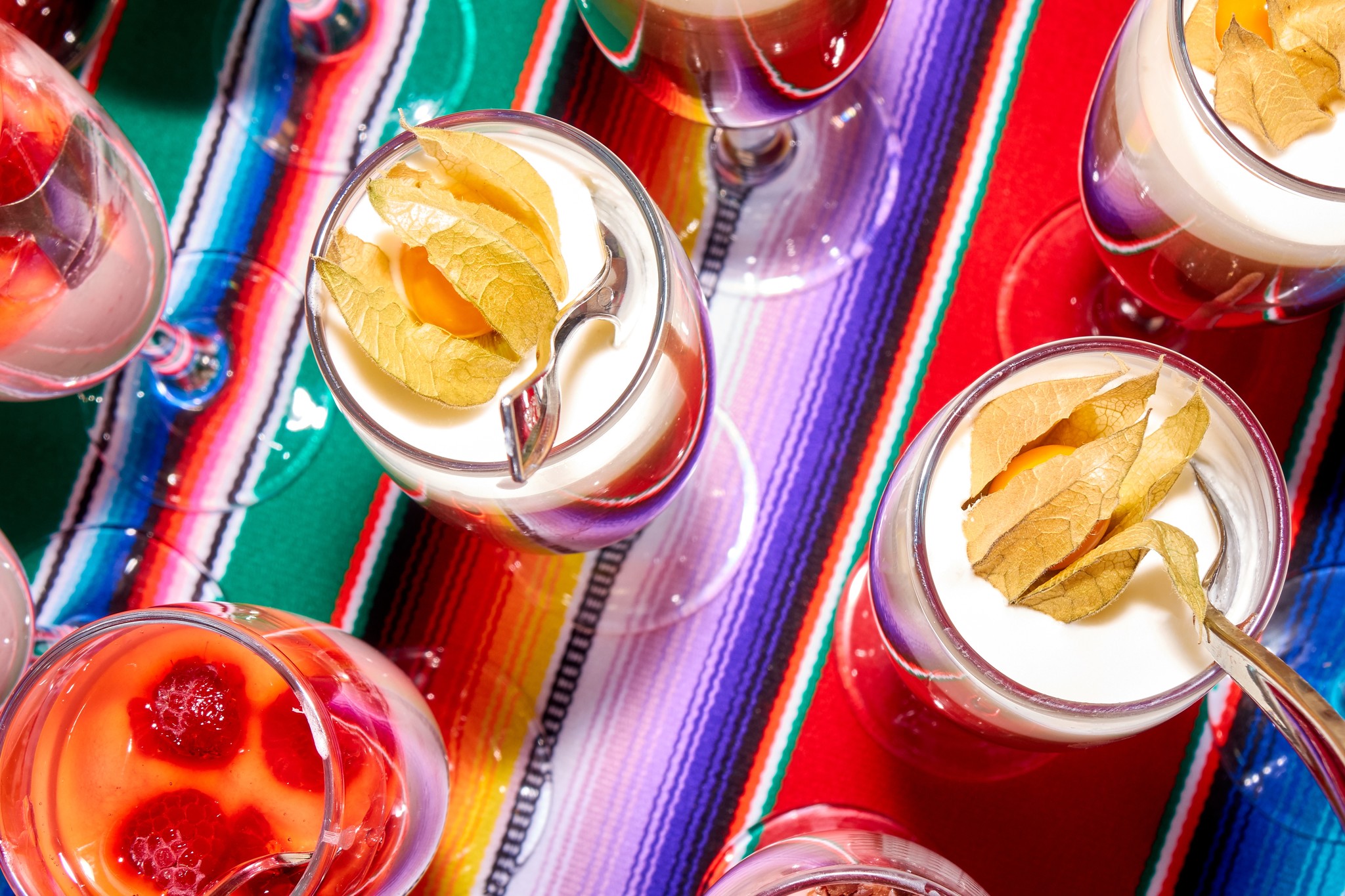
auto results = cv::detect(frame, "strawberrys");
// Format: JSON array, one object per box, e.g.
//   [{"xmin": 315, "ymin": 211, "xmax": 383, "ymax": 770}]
[
  {"xmin": 105, "ymin": 658, "xmax": 397, "ymax": 896},
  {"xmin": 0, "ymin": 121, "xmax": 70, "ymax": 304}
]
